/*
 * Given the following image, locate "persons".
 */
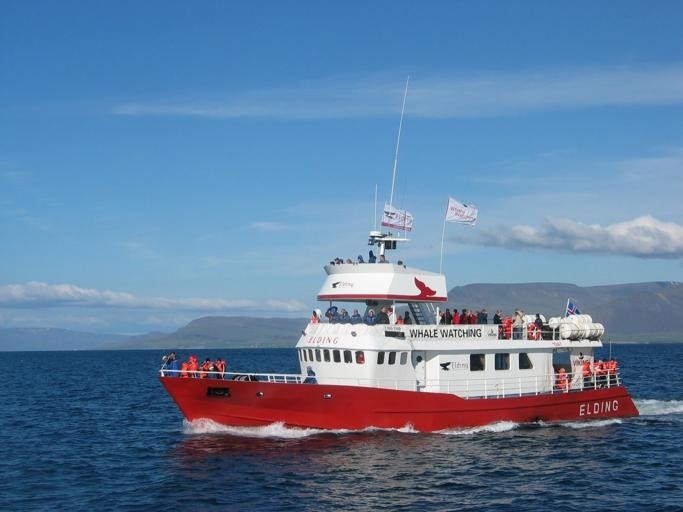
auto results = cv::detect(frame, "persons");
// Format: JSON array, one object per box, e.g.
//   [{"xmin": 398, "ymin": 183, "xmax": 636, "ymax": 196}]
[
  {"xmin": 398, "ymin": 261, "xmax": 406, "ymax": 268},
  {"xmin": 311, "ymin": 305, "xmax": 412, "ymax": 325},
  {"xmin": 162, "ymin": 352, "xmax": 226, "ymax": 378},
  {"xmin": 330, "ymin": 258, "xmax": 343, "ymax": 265},
  {"xmin": 358, "ymin": 255, "xmax": 364, "ymax": 263},
  {"xmin": 554, "ymin": 352, "xmax": 620, "ymax": 389},
  {"xmin": 535, "ymin": 313, "xmax": 543, "ymax": 340},
  {"xmin": 357, "ymin": 353, "xmax": 364, "ymax": 363},
  {"xmin": 303, "ymin": 366, "xmax": 318, "ymax": 384},
  {"xmin": 379, "ymin": 255, "xmax": 389, "ymax": 263},
  {"xmin": 369, "ymin": 250, "xmax": 376, "ymax": 263},
  {"xmin": 347, "ymin": 259, "xmax": 352, "ymax": 263},
  {"xmin": 439, "ymin": 309, "xmax": 525, "ymax": 339}
]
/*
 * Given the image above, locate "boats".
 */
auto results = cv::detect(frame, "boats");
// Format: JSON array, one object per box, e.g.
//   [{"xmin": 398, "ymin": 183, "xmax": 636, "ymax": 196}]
[{"xmin": 157, "ymin": 232, "xmax": 640, "ymax": 433}]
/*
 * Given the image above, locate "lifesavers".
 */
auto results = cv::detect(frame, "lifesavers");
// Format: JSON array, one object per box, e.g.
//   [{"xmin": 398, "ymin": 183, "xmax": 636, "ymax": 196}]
[{"xmin": 528, "ymin": 324, "xmax": 541, "ymax": 340}]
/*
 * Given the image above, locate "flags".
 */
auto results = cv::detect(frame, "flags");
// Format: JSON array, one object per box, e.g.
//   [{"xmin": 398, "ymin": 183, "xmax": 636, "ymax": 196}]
[
  {"xmin": 446, "ymin": 197, "xmax": 478, "ymax": 225},
  {"xmin": 380, "ymin": 204, "xmax": 415, "ymax": 232},
  {"xmin": 566, "ymin": 302, "xmax": 580, "ymax": 317}
]
